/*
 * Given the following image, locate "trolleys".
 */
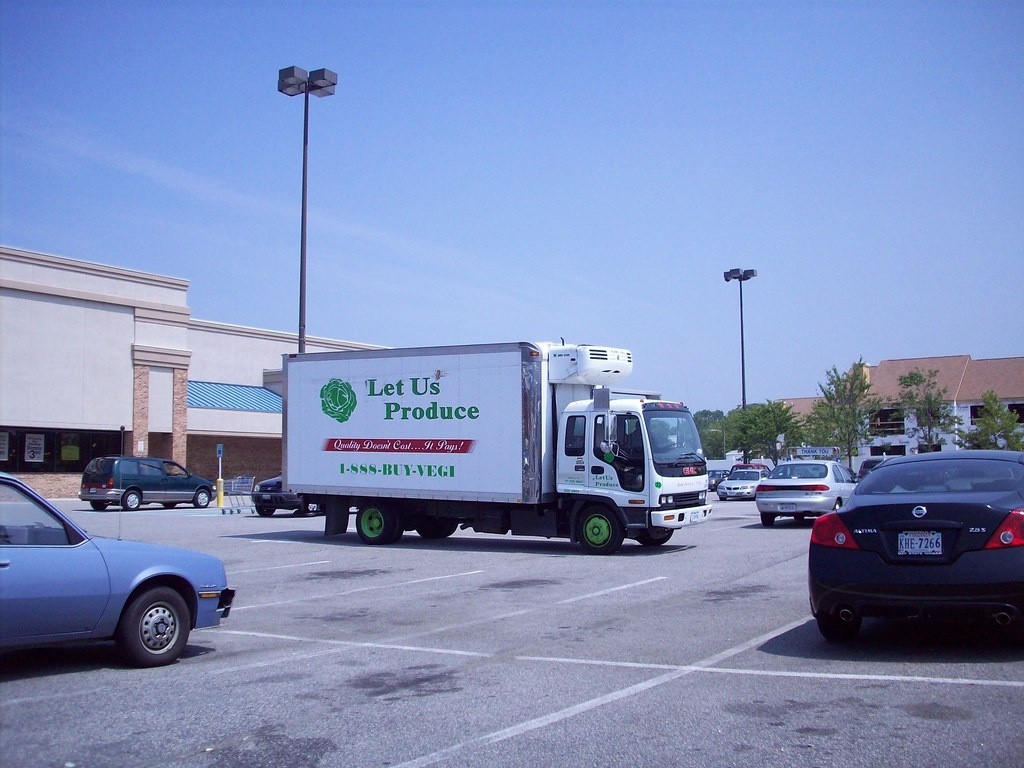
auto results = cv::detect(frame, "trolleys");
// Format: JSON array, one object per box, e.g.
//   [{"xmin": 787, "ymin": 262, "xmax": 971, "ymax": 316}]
[{"xmin": 221, "ymin": 474, "xmax": 257, "ymax": 516}]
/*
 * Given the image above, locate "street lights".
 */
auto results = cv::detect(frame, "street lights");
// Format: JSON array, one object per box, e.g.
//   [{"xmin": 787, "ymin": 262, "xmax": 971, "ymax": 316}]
[
  {"xmin": 723, "ymin": 268, "xmax": 757, "ymax": 466},
  {"xmin": 118, "ymin": 425, "xmax": 126, "ymax": 541},
  {"xmin": 276, "ymin": 66, "xmax": 338, "ymax": 352}
]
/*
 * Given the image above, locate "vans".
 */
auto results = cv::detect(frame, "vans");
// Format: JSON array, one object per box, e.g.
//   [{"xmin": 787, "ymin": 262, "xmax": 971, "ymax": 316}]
[{"xmin": 77, "ymin": 455, "xmax": 217, "ymax": 512}]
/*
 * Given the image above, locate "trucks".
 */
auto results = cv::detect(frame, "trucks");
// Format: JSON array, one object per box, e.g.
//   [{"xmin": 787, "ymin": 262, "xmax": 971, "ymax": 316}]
[{"xmin": 281, "ymin": 338, "xmax": 714, "ymax": 555}]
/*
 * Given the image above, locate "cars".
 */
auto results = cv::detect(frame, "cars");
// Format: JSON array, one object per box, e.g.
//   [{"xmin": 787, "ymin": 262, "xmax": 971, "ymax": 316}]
[
  {"xmin": 717, "ymin": 469, "xmax": 768, "ymax": 501},
  {"xmin": 858, "ymin": 454, "xmax": 902, "ymax": 482},
  {"xmin": 729, "ymin": 463, "xmax": 771, "ymax": 478},
  {"xmin": 808, "ymin": 450, "xmax": 1024, "ymax": 643},
  {"xmin": 251, "ymin": 471, "xmax": 322, "ymax": 518},
  {"xmin": 755, "ymin": 460, "xmax": 859, "ymax": 526},
  {"xmin": 707, "ymin": 469, "xmax": 730, "ymax": 492},
  {"xmin": 0, "ymin": 471, "xmax": 236, "ymax": 669}
]
